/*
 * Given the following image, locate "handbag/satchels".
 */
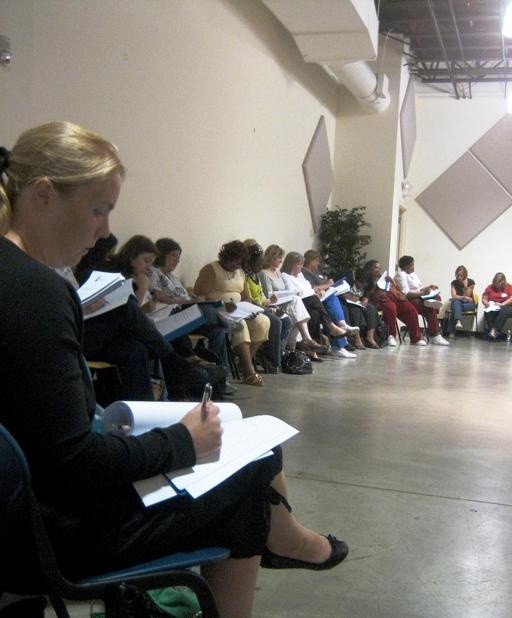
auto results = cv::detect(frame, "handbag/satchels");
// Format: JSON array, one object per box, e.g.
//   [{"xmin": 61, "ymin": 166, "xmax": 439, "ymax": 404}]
[
  {"xmin": 281, "ymin": 350, "xmax": 312, "ymax": 375},
  {"xmin": 175, "ymin": 364, "xmax": 230, "ymax": 402}
]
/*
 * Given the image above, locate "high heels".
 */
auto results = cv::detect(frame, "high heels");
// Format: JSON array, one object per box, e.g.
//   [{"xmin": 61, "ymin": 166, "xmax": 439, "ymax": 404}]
[
  {"xmin": 365, "ymin": 340, "xmax": 382, "ymax": 349},
  {"xmin": 307, "ymin": 354, "xmax": 322, "ymax": 362},
  {"xmin": 353, "ymin": 342, "xmax": 366, "ymax": 349}
]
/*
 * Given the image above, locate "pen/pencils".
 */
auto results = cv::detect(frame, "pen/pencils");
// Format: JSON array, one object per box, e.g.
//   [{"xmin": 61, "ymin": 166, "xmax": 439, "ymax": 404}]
[
  {"xmin": 230, "ymin": 298, "xmax": 233, "ymax": 302},
  {"xmin": 190, "ymin": 291, "xmax": 198, "ymax": 299},
  {"xmin": 200, "ymin": 382, "xmax": 212, "ymax": 411}
]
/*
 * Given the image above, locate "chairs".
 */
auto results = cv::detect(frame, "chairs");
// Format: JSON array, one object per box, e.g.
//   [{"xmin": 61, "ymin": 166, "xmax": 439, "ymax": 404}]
[
  {"xmin": 0, "ymin": 423, "xmax": 233, "ymax": 618},
  {"xmin": 445, "ymin": 291, "xmax": 480, "ymax": 337}
]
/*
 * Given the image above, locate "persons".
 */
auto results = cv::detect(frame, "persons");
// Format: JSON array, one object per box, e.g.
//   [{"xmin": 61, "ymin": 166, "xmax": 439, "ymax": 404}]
[
  {"xmin": 395, "ymin": 256, "xmax": 449, "ymax": 345},
  {"xmin": 447, "ymin": 266, "xmax": 476, "ymax": 339},
  {"xmin": 334, "ymin": 272, "xmax": 380, "ymax": 350},
  {"xmin": 481, "ymin": 273, "xmax": 512, "ymax": 341},
  {"xmin": 0, "ymin": 147, "xmax": 228, "ymax": 400},
  {"xmin": 81, "ymin": 234, "xmax": 161, "ymax": 310},
  {"xmin": 302, "ymin": 251, "xmax": 359, "ymax": 358},
  {"xmin": 0, "ymin": 122, "xmax": 349, "ymax": 618},
  {"xmin": 280, "ymin": 252, "xmax": 350, "ymax": 362},
  {"xmin": 194, "ymin": 242, "xmax": 271, "ymax": 386},
  {"xmin": 355, "ymin": 260, "xmax": 427, "ymax": 346},
  {"xmin": 246, "ymin": 245, "xmax": 293, "ymax": 374},
  {"xmin": 74, "ymin": 232, "xmax": 119, "ymax": 286},
  {"xmin": 259, "ymin": 246, "xmax": 327, "ymax": 362},
  {"xmin": 149, "ymin": 239, "xmax": 243, "ymax": 395}
]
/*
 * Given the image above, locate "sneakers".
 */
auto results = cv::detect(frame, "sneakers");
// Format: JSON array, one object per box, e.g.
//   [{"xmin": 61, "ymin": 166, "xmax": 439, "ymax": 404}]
[
  {"xmin": 388, "ymin": 335, "xmax": 396, "ymax": 346},
  {"xmin": 225, "ymin": 380, "xmax": 236, "ymax": 394},
  {"xmin": 410, "ymin": 340, "xmax": 427, "ymax": 346}
]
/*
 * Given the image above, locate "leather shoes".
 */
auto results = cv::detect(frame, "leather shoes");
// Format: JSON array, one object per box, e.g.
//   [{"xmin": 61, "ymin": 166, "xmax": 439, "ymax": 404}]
[
  {"xmin": 345, "ymin": 325, "xmax": 359, "ymax": 334},
  {"xmin": 301, "ymin": 340, "xmax": 323, "ymax": 349},
  {"xmin": 260, "ymin": 534, "xmax": 347, "ymax": 571},
  {"xmin": 431, "ymin": 335, "xmax": 449, "ymax": 345},
  {"xmin": 331, "ymin": 329, "xmax": 351, "ymax": 338},
  {"xmin": 337, "ymin": 351, "xmax": 356, "ymax": 358}
]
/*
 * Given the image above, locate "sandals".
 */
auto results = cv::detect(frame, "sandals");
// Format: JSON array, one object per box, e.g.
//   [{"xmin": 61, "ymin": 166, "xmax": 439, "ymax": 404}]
[{"xmin": 244, "ymin": 374, "xmax": 265, "ymax": 386}]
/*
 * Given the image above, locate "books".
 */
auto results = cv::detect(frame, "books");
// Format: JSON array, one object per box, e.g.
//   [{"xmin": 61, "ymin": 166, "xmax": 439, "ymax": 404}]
[
  {"xmin": 99, "ymin": 386, "xmax": 300, "ymax": 509},
  {"xmin": 483, "ymin": 303, "xmax": 501, "ymax": 314},
  {"xmin": 344, "ymin": 294, "xmax": 368, "ymax": 310},
  {"xmin": 315, "ymin": 287, "xmax": 337, "ymax": 302},
  {"xmin": 218, "ymin": 300, "xmax": 265, "ymax": 325},
  {"xmin": 77, "ymin": 272, "xmax": 135, "ymax": 322},
  {"xmin": 419, "ymin": 288, "xmax": 441, "ymax": 299},
  {"xmin": 376, "ymin": 269, "xmax": 391, "ymax": 293},
  {"xmin": 332, "ymin": 279, "xmax": 351, "ymax": 297},
  {"xmin": 155, "ymin": 302, "xmax": 207, "ymax": 343},
  {"xmin": 268, "ymin": 289, "xmax": 294, "ymax": 306},
  {"xmin": 300, "ymin": 289, "xmax": 316, "ymax": 300}
]
